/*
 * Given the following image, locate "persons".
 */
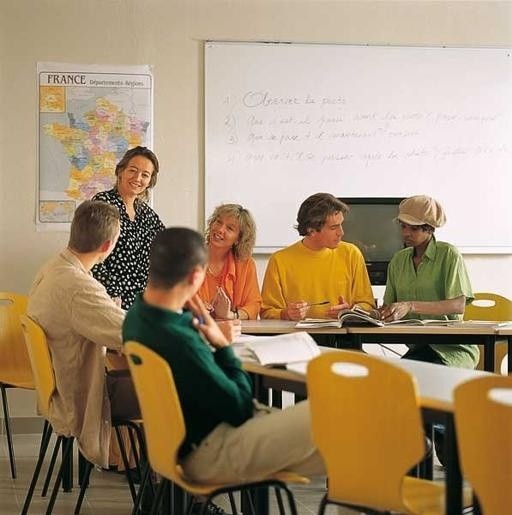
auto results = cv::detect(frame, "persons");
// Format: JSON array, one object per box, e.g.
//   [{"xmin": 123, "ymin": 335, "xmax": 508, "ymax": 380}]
[
  {"xmin": 89, "ymin": 145, "xmax": 167, "ymax": 311},
  {"xmin": 258, "ymin": 193, "xmax": 376, "ymax": 406},
  {"xmin": 26, "ymin": 200, "xmax": 230, "ymax": 515},
  {"xmin": 121, "ymin": 226, "xmax": 368, "ymax": 515},
  {"xmin": 372, "ymin": 195, "xmax": 480, "ymax": 477}
]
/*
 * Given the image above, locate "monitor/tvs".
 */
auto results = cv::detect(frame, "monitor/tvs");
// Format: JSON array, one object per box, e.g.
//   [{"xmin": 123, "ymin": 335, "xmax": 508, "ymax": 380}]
[{"xmin": 336, "ymin": 197, "xmax": 407, "ymax": 285}]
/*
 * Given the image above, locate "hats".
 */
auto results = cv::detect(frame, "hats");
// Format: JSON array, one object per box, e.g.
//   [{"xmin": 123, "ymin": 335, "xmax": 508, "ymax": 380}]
[{"xmin": 393, "ymin": 195, "xmax": 446, "ymax": 229}]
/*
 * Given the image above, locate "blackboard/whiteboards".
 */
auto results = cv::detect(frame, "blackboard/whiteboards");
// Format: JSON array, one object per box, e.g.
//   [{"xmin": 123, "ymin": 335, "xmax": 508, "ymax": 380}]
[{"xmin": 202, "ymin": 39, "xmax": 512, "ymax": 255}]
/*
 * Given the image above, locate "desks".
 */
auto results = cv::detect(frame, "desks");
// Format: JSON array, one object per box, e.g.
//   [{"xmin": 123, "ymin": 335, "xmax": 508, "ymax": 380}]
[
  {"xmin": 241, "ymin": 318, "xmax": 348, "ymax": 351},
  {"xmin": 348, "ymin": 321, "xmax": 512, "ymax": 375}
]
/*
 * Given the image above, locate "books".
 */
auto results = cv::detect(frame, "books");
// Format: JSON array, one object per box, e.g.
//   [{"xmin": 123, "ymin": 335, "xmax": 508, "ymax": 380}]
[
  {"xmin": 384, "ymin": 319, "xmax": 459, "ymax": 326},
  {"xmin": 293, "ymin": 308, "xmax": 383, "ymax": 328}
]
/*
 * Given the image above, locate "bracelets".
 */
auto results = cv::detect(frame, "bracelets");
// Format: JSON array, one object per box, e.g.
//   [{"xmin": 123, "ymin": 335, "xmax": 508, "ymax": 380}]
[{"xmin": 231, "ymin": 310, "xmax": 239, "ymax": 319}]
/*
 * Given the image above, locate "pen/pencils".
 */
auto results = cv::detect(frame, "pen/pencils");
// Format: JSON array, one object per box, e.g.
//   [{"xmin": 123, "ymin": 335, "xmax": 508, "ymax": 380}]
[{"xmin": 307, "ymin": 302, "xmax": 330, "ymax": 306}]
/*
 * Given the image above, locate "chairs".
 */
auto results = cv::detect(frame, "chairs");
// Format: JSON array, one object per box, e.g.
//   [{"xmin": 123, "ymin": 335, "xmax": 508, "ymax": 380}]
[
  {"xmin": 305, "ymin": 350, "xmax": 473, "ymax": 515},
  {"xmin": 453, "ymin": 375, "xmax": 512, "ymax": 515},
  {"xmin": 124, "ymin": 341, "xmax": 310, "ymax": 515},
  {"xmin": 0, "ymin": 292, "xmax": 39, "ymax": 479},
  {"xmin": 15, "ymin": 314, "xmax": 155, "ymax": 514},
  {"xmin": 463, "ymin": 293, "xmax": 512, "ymax": 375}
]
